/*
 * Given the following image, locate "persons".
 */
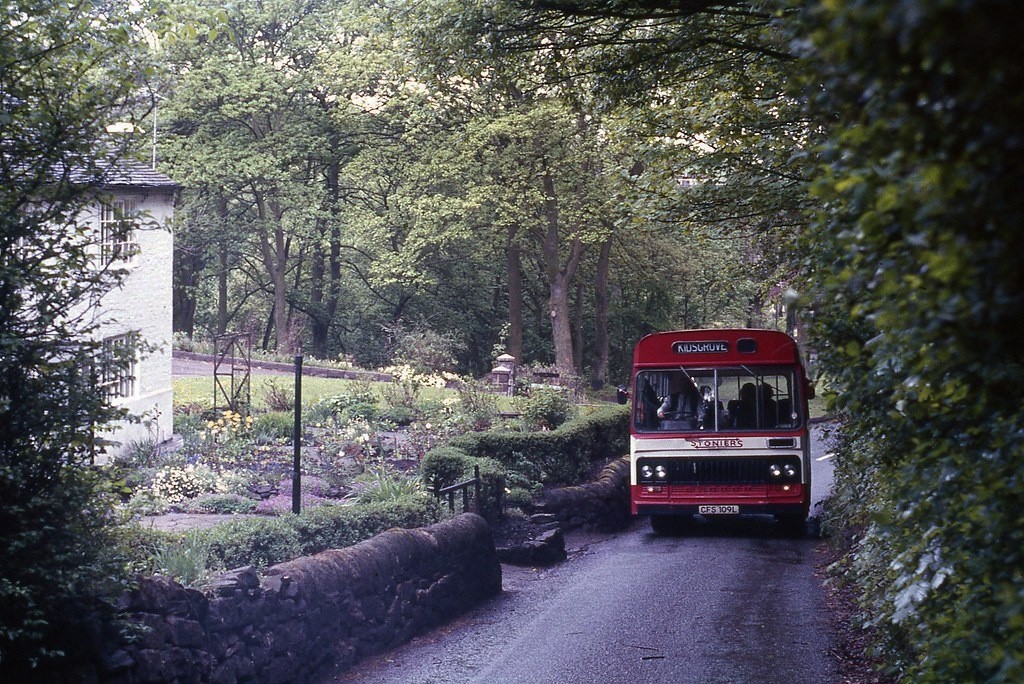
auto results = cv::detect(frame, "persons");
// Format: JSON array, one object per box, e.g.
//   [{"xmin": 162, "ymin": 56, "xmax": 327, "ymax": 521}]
[
  {"xmin": 657, "ymin": 378, "xmax": 706, "ymax": 423},
  {"xmin": 710, "ymin": 382, "xmax": 784, "ymax": 430}
]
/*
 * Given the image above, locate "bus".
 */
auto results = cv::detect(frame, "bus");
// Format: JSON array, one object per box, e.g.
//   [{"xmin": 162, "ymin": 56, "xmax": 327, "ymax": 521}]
[{"xmin": 616, "ymin": 327, "xmax": 815, "ymax": 537}]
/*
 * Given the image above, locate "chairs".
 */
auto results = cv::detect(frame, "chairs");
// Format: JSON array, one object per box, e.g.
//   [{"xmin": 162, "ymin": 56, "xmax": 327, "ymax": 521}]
[{"xmin": 661, "ymin": 397, "xmax": 799, "ymax": 431}]
